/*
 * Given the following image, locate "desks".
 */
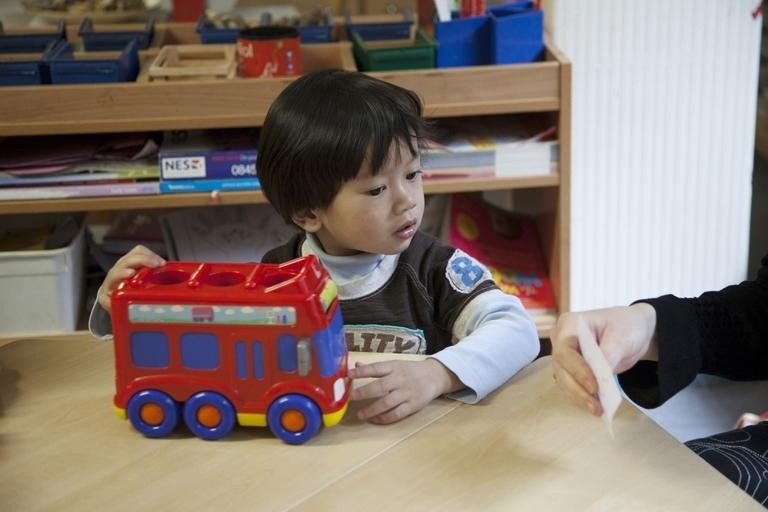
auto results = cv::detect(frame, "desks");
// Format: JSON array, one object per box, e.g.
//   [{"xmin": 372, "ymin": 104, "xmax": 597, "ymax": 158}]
[{"xmin": 1, "ymin": 328, "xmax": 766, "ymax": 511}]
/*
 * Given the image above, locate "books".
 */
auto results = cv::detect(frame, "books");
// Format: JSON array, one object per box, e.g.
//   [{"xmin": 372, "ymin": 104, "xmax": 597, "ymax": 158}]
[
  {"xmin": 415, "ymin": 116, "xmax": 558, "ymax": 182},
  {"xmin": 1, "ymin": 127, "xmax": 262, "ymax": 201},
  {"xmin": 439, "ymin": 193, "xmax": 560, "ymax": 331}
]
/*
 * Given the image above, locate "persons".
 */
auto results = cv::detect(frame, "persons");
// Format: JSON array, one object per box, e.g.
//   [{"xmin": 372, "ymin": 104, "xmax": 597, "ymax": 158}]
[
  {"xmin": 549, "ymin": 256, "xmax": 768, "ymax": 512},
  {"xmin": 88, "ymin": 69, "xmax": 540, "ymax": 425}
]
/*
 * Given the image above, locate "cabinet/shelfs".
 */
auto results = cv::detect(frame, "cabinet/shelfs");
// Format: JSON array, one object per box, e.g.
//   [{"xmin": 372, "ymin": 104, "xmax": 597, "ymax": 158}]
[{"xmin": 0, "ymin": 0, "xmax": 571, "ymax": 335}]
